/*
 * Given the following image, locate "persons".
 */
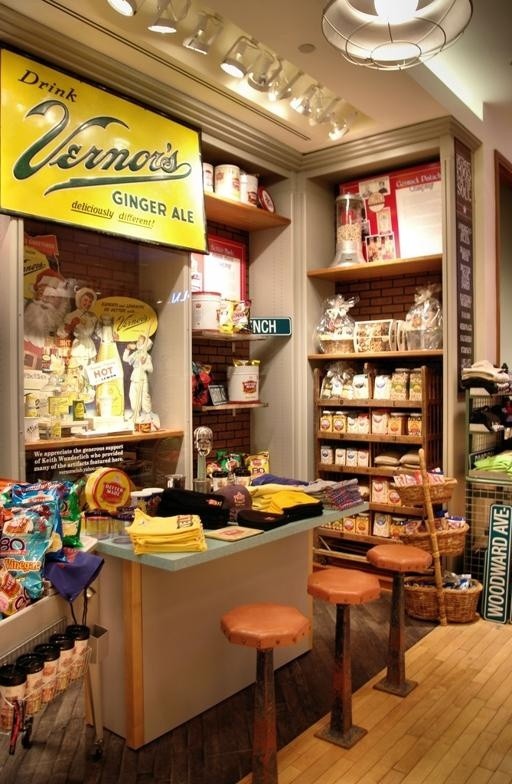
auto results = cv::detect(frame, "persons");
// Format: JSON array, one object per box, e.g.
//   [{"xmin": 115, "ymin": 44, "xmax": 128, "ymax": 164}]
[
  {"xmin": 57, "ymin": 286, "xmax": 118, "ymax": 409},
  {"xmin": 124, "ymin": 335, "xmax": 151, "ymax": 421}
]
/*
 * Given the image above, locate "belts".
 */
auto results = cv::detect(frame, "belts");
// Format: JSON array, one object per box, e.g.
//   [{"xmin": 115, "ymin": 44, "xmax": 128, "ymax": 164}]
[{"xmin": 25, "ymin": 349, "xmax": 47, "ymax": 369}]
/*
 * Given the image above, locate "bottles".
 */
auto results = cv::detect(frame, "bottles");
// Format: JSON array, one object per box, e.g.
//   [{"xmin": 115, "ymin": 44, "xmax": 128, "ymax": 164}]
[
  {"xmin": 202, "ymin": 163, "xmax": 213, "ymax": 193},
  {"xmin": 322, "ymin": 523, "xmax": 332, "ymax": 529},
  {"xmin": 240, "ymin": 175, "xmax": 257, "ymax": 205},
  {"xmin": 214, "ymin": 165, "xmax": 240, "ymax": 200},
  {"xmin": 388, "ymin": 413, "xmax": 406, "ymax": 434},
  {"xmin": 320, "ymin": 411, "xmax": 333, "ymax": 431},
  {"xmin": 111, "ymin": 512, "xmax": 134, "ymax": 545},
  {"xmin": 390, "ymin": 517, "xmax": 407, "ymax": 538},
  {"xmin": 84, "ymin": 511, "xmax": 111, "ymax": 540},
  {"xmin": 356, "ymin": 514, "xmax": 369, "ymax": 534},
  {"xmin": 408, "ymin": 414, "xmax": 422, "ymax": 436},
  {"xmin": 235, "ymin": 470, "xmax": 251, "ymax": 486},
  {"xmin": 227, "ymin": 366, "xmax": 259, "ymax": 403},
  {"xmin": 95, "ymin": 315, "xmax": 124, "ymax": 418},
  {"xmin": 191, "ymin": 291, "xmax": 221, "ymax": 334},
  {"xmin": 342, "ymin": 516, "xmax": 356, "ymax": 534},
  {"xmin": 332, "ymin": 520, "xmax": 343, "ymax": 532},
  {"xmin": 333, "ymin": 412, "xmax": 349, "ymax": 433}
]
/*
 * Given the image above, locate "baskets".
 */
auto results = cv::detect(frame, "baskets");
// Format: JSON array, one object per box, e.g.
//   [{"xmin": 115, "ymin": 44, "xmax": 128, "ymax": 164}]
[
  {"xmin": 392, "ymin": 477, "xmax": 457, "ymax": 506},
  {"xmin": 404, "ymin": 575, "xmax": 483, "ymax": 623}
]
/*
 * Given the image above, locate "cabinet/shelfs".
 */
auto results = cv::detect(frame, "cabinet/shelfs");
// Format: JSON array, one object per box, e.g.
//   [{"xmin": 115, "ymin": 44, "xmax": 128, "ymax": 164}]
[
  {"xmin": 0, "ymin": 46, "xmax": 209, "ymax": 523},
  {"xmin": 301, "ymin": 111, "xmax": 484, "ymax": 577},
  {"xmin": 139, "ymin": 131, "xmax": 300, "ymax": 489}
]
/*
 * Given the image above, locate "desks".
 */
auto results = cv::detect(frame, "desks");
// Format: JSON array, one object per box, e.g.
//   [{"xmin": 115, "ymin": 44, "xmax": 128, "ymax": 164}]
[{"xmin": 85, "ymin": 470, "xmax": 372, "ymax": 754}]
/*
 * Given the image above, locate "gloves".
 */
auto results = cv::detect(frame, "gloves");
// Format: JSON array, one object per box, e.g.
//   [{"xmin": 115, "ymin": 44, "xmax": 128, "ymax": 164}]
[{"xmin": 66, "ymin": 318, "xmax": 81, "ymax": 336}]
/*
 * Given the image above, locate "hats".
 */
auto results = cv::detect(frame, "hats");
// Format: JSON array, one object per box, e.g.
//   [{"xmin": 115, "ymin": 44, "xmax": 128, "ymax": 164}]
[
  {"xmin": 34, "ymin": 268, "xmax": 69, "ymax": 293},
  {"xmin": 138, "ymin": 335, "xmax": 153, "ymax": 351},
  {"xmin": 75, "ymin": 288, "xmax": 97, "ymax": 310}
]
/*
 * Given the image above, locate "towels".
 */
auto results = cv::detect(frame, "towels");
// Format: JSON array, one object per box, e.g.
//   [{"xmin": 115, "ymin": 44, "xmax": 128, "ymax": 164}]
[{"xmin": 399, "ymin": 519, "xmax": 470, "ymax": 557}]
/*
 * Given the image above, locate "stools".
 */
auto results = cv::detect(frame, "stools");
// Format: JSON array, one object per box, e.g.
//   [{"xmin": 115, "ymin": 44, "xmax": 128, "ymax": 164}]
[
  {"xmin": 219, "ymin": 603, "xmax": 312, "ymax": 783},
  {"xmin": 306, "ymin": 566, "xmax": 382, "ymax": 749},
  {"xmin": 367, "ymin": 540, "xmax": 433, "ymax": 696}
]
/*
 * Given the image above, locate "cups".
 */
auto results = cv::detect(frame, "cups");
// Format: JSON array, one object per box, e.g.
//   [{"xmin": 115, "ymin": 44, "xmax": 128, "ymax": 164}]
[
  {"xmin": 130, "ymin": 490, "xmax": 153, "ymax": 518},
  {"xmin": 142, "ymin": 487, "xmax": 164, "ymax": 516},
  {"xmin": 233, "ymin": 466, "xmax": 251, "ymax": 489},
  {"xmin": 24, "ymin": 416, "xmax": 40, "ymax": 442}
]
